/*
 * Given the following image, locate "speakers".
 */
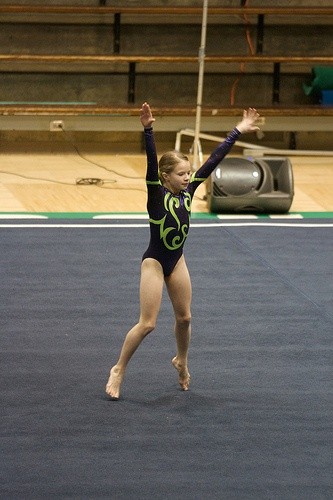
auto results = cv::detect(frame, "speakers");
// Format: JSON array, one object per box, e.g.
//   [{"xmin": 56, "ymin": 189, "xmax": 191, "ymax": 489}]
[{"xmin": 207, "ymin": 157, "xmax": 295, "ymax": 214}]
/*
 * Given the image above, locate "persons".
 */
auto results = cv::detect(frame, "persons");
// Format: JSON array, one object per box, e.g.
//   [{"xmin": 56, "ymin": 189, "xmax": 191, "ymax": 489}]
[{"xmin": 100, "ymin": 101, "xmax": 260, "ymax": 407}]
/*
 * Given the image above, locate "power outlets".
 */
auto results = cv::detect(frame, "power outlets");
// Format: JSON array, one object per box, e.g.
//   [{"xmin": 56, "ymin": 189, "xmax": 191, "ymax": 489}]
[{"xmin": 49, "ymin": 120, "xmax": 65, "ymax": 132}]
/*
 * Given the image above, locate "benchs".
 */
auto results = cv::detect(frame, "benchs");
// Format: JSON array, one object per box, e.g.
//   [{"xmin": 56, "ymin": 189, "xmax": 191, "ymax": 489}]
[{"xmin": 0, "ymin": 0, "xmax": 332, "ymax": 134}]
